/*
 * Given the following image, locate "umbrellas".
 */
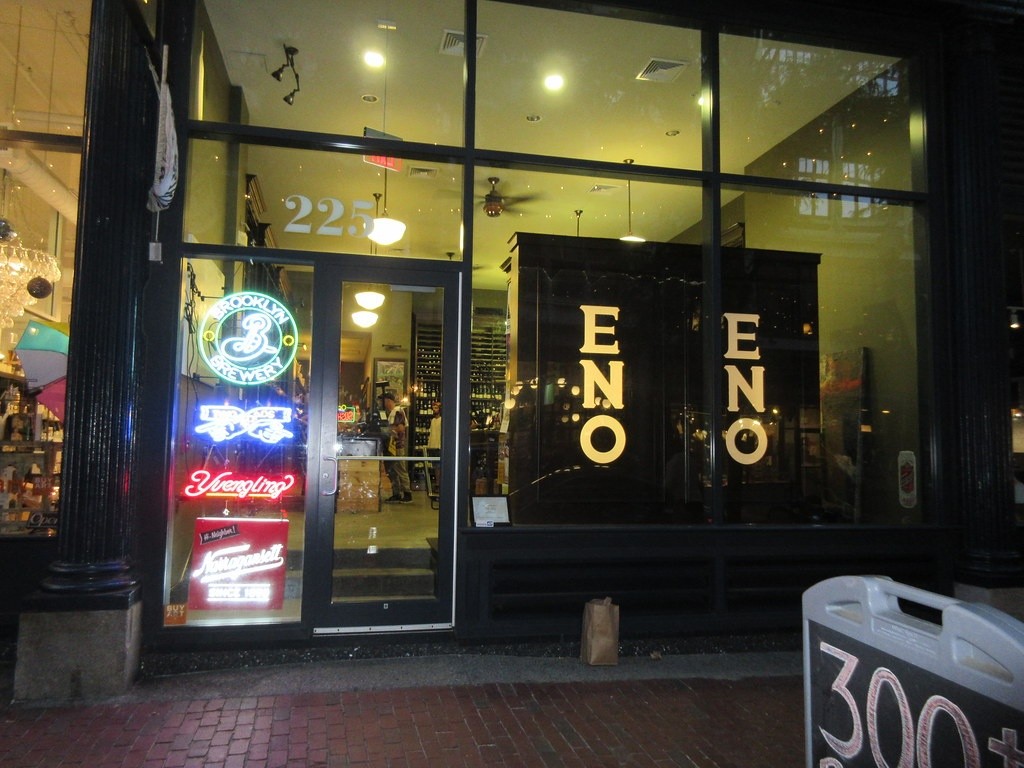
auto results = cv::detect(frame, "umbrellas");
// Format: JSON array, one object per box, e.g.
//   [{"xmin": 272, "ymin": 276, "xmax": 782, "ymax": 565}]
[{"xmin": 15, "ymin": 318, "xmax": 69, "ymax": 423}]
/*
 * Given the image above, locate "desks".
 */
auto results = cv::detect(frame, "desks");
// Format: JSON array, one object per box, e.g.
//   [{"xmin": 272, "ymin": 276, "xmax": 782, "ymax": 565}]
[{"xmin": 470, "ymin": 440, "xmax": 500, "ymax": 498}]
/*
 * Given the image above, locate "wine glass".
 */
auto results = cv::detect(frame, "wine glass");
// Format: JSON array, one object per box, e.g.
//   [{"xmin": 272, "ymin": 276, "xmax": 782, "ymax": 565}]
[{"xmin": 0, "ymin": 244, "xmax": 61, "ymax": 326}]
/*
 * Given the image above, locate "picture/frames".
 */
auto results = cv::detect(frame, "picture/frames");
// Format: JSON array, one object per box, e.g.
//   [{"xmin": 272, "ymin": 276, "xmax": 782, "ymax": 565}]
[{"xmin": 373, "ymin": 357, "xmax": 408, "ymax": 414}]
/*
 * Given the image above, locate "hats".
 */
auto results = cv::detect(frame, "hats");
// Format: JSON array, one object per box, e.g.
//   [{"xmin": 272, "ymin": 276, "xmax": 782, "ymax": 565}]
[
  {"xmin": 378, "ymin": 393, "xmax": 395, "ymax": 401},
  {"xmin": 432, "ymin": 402, "xmax": 442, "ymax": 409}
]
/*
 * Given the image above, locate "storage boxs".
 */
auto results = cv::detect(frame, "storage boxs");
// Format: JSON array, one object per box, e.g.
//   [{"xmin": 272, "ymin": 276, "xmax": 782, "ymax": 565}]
[{"xmin": 335, "ymin": 459, "xmax": 381, "ymax": 515}]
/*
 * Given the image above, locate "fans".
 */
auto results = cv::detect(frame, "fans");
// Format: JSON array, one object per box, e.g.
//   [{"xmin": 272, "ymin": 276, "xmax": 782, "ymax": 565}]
[{"xmin": 435, "ymin": 176, "xmax": 545, "ymax": 217}]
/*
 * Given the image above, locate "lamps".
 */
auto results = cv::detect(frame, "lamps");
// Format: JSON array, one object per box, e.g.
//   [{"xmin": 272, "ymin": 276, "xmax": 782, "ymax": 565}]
[
  {"xmin": 283, "ymin": 54, "xmax": 300, "ymax": 106},
  {"xmin": 270, "ymin": 43, "xmax": 299, "ymax": 81},
  {"xmin": 353, "ymin": 192, "xmax": 385, "ymax": 312},
  {"xmin": 362, "ymin": 18, "xmax": 407, "ymax": 247},
  {"xmin": 0, "ymin": 171, "xmax": 62, "ymax": 332},
  {"xmin": 481, "ymin": 202, "xmax": 504, "ymax": 217},
  {"xmin": 350, "ymin": 310, "xmax": 378, "ymax": 328},
  {"xmin": 620, "ymin": 158, "xmax": 647, "ymax": 241}
]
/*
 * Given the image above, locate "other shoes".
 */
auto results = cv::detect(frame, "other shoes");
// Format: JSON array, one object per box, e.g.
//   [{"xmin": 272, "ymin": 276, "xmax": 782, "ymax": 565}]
[
  {"xmin": 384, "ymin": 494, "xmax": 402, "ymax": 502},
  {"xmin": 400, "ymin": 492, "xmax": 413, "ymax": 503}
]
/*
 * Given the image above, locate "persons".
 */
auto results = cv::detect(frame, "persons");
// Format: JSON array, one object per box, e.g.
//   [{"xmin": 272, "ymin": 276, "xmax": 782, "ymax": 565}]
[
  {"xmin": 377, "ymin": 393, "xmax": 413, "ymax": 504},
  {"xmin": 5, "ymin": 399, "xmax": 35, "ymax": 508},
  {"xmin": 469, "ymin": 410, "xmax": 486, "ymax": 498},
  {"xmin": 426, "ymin": 402, "xmax": 441, "ymax": 494}
]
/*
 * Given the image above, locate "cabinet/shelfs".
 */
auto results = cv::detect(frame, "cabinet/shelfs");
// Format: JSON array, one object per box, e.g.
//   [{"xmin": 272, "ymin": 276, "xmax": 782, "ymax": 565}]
[
  {"xmin": 0, "ymin": 371, "xmax": 38, "ymax": 456},
  {"xmin": 505, "ymin": 230, "xmax": 830, "ymax": 525},
  {"xmin": 0, "ymin": 440, "xmax": 63, "ymax": 538},
  {"xmin": 663, "ymin": 293, "xmax": 830, "ymax": 522},
  {"xmin": 407, "ymin": 311, "xmax": 506, "ymax": 493}
]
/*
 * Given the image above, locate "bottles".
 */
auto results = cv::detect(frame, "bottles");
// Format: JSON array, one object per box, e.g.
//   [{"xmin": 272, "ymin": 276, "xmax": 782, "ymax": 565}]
[
  {"xmin": 472, "ymin": 464, "xmax": 500, "ymax": 497},
  {"xmin": 415, "ymin": 416, "xmax": 431, "ymax": 446},
  {"xmin": 414, "ymin": 462, "xmax": 436, "ymax": 490},
  {"xmin": 471, "ymin": 401, "xmax": 500, "ymax": 429},
  {"xmin": 416, "ymin": 401, "xmax": 438, "ymax": 414},
  {"xmin": 415, "ymin": 348, "xmax": 504, "ymax": 399}
]
